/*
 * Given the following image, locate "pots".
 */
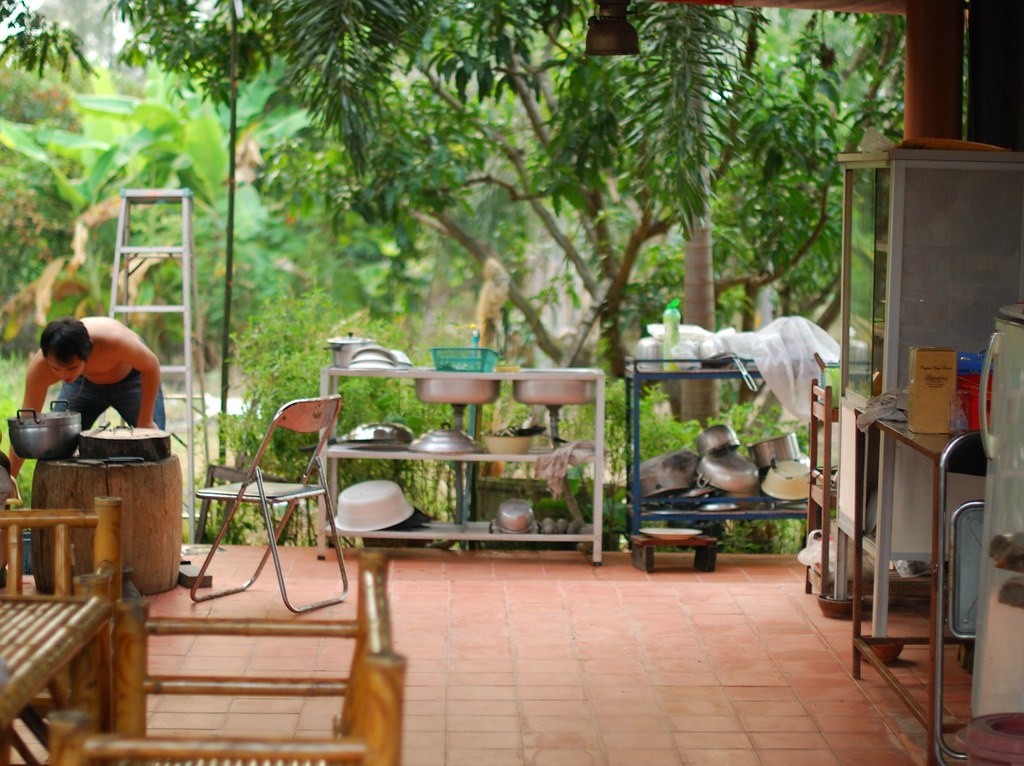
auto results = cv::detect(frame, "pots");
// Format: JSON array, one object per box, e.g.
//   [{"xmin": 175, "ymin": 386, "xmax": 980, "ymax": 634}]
[
  {"xmin": 624, "ymin": 329, "xmax": 826, "ymax": 517},
  {"xmin": 7, "ymin": 400, "xmax": 82, "ymax": 460}
]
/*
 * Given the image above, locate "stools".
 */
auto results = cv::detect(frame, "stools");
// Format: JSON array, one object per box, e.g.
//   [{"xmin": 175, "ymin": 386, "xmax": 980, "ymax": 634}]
[{"xmin": 632, "ymin": 534, "xmax": 718, "ymax": 573}]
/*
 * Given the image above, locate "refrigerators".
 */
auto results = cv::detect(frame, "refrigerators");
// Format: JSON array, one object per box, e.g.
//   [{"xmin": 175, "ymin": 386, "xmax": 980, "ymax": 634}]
[{"xmin": 972, "ymin": 303, "xmax": 1024, "ymax": 730}]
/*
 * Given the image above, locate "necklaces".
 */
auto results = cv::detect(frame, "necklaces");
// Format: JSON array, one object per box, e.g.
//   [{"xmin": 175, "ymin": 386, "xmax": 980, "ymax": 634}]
[{"xmin": 61, "ymin": 372, "xmax": 85, "ymax": 400}]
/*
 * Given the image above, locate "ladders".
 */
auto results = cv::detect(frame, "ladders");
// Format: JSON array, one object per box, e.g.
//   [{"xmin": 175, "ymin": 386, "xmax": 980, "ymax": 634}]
[{"xmin": 107, "ymin": 187, "xmax": 209, "ymax": 543}]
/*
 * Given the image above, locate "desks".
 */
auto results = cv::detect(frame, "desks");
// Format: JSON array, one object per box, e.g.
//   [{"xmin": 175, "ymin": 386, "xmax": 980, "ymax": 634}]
[{"xmin": 853, "ymin": 407, "xmax": 986, "ymax": 766}]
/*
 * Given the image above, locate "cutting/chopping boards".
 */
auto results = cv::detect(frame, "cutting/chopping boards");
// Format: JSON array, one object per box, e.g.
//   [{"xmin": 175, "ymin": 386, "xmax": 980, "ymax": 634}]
[{"xmin": 78, "ymin": 429, "xmax": 172, "ymax": 462}]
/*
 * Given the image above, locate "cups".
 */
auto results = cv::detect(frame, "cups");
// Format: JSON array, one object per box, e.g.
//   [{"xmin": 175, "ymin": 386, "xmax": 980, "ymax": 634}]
[{"xmin": 947, "ymin": 389, "xmax": 971, "ymax": 441}]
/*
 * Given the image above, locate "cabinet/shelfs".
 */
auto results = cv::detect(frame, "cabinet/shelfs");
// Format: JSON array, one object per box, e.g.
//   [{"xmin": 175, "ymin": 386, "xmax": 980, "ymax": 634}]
[
  {"xmin": 821, "ymin": 149, "xmax": 1023, "ymax": 662},
  {"xmin": 319, "ymin": 364, "xmax": 604, "ymax": 565},
  {"xmin": 625, "ymin": 346, "xmax": 838, "ymax": 597}
]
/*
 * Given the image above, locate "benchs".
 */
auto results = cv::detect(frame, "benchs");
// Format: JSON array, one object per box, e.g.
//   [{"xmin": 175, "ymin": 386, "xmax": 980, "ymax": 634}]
[{"xmin": 0, "ymin": 494, "xmax": 120, "ymax": 765}]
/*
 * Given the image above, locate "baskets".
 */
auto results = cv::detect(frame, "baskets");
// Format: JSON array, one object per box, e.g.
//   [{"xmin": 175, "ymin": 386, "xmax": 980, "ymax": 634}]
[{"xmin": 430, "ymin": 346, "xmax": 499, "ymax": 373}]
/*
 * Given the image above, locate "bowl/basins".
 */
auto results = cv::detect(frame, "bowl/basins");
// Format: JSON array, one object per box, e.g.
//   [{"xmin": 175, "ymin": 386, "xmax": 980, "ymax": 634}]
[
  {"xmin": 333, "ymin": 340, "xmax": 597, "ymax": 532},
  {"xmin": 818, "ymin": 593, "xmax": 864, "ymax": 618},
  {"xmin": 856, "ymin": 636, "xmax": 905, "ymax": 663}
]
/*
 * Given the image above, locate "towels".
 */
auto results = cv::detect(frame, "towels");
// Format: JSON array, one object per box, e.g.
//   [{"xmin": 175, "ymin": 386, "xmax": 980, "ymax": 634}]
[{"xmin": 857, "ymin": 385, "xmax": 910, "ymax": 433}]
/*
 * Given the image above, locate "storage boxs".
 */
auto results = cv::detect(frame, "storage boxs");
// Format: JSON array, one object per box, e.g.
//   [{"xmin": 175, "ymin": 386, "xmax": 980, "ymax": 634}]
[{"xmin": 907, "ymin": 345, "xmax": 958, "ymax": 432}]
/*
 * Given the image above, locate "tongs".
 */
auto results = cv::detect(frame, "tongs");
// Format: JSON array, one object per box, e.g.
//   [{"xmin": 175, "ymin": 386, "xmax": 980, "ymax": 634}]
[{"xmin": 113, "ymin": 425, "xmax": 135, "ymax": 432}]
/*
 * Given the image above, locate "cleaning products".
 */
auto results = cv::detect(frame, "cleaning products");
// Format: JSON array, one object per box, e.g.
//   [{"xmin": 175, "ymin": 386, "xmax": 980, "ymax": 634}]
[{"xmin": 661, "ymin": 297, "xmax": 681, "ymax": 372}]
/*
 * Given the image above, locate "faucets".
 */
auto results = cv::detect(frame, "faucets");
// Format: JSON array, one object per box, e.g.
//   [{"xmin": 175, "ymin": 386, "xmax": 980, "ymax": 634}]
[{"xmin": 472, "ymin": 329, "xmax": 480, "ymax": 344}]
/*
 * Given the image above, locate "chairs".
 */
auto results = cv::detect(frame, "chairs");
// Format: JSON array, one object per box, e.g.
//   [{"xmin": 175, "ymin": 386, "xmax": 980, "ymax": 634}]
[{"xmin": 189, "ymin": 393, "xmax": 349, "ymax": 615}]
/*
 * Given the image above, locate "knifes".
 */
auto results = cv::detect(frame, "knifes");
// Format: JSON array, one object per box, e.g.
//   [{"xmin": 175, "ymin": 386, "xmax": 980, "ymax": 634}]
[{"xmin": 87, "ymin": 421, "xmax": 115, "ymax": 438}]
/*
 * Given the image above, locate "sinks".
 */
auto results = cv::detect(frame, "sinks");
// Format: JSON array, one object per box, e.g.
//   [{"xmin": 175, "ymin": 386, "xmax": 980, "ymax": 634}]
[
  {"xmin": 512, "ymin": 367, "xmax": 597, "ymax": 406},
  {"xmin": 414, "ymin": 367, "xmax": 501, "ymax": 404}
]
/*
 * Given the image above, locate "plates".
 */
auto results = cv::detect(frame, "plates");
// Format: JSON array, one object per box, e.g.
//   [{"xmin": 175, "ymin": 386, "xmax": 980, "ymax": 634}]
[{"xmin": 639, "ymin": 528, "xmax": 703, "ymax": 539}]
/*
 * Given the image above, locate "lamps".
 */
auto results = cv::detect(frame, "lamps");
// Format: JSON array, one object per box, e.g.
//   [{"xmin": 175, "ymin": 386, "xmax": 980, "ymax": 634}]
[{"xmin": 583, "ymin": 1, "xmax": 642, "ymax": 59}]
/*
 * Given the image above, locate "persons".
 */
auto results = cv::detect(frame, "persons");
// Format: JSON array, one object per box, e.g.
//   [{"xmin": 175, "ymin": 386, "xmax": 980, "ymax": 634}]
[{"xmin": 8, "ymin": 315, "xmax": 167, "ymax": 498}]
